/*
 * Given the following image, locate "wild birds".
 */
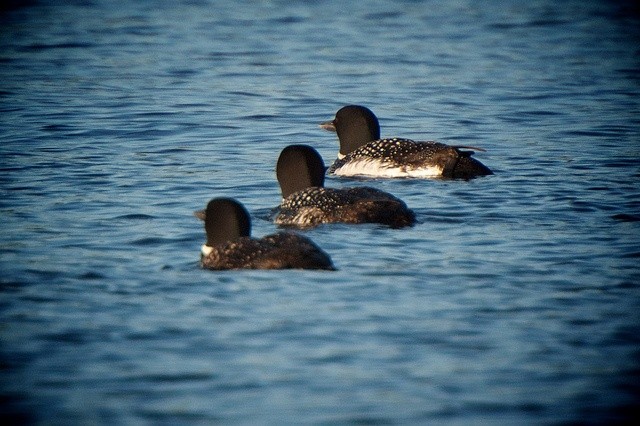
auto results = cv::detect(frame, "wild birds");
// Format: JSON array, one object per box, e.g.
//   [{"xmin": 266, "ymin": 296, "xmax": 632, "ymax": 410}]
[
  {"xmin": 267, "ymin": 142, "xmax": 424, "ymax": 232},
  {"xmin": 193, "ymin": 195, "xmax": 337, "ymax": 274},
  {"xmin": 318, "ymin": 103, "xmax": 494, "ymax": 183}
]
